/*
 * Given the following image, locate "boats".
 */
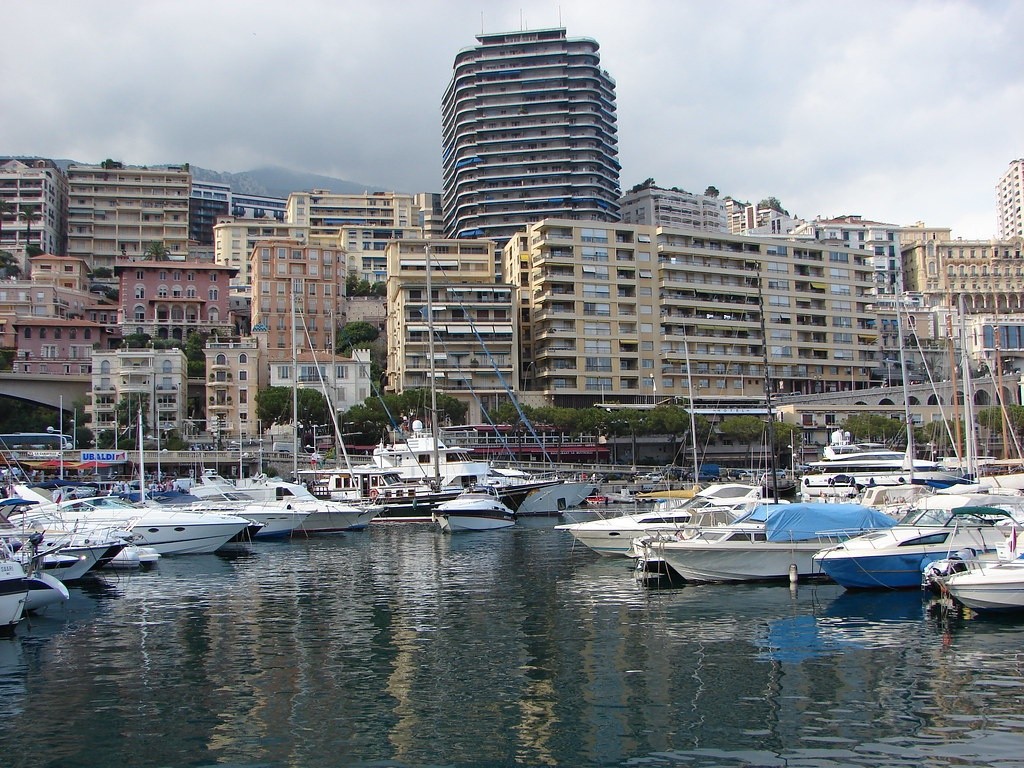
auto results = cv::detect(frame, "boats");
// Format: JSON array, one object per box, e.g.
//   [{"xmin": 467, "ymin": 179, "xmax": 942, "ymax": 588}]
[
  {"xmin": 818, "ymin": 587, "xmax": 940, "ymax": 620},
  {"xmin": 0, "ymin": 240, "xmax": 1024, "ymax": 635}
]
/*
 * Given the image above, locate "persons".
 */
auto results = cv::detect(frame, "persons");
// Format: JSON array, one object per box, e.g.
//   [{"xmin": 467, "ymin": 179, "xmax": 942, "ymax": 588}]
[
  {"xmin": 306, "ymin": 480, "xmax": 314, "ymax": 494},
  {"xmin": 31, "ymin": 468, "xmax": 39, "ymax": 481},
  {"xmin": 249, "ymin": 438, "xmax": 253, "ymax": 444},
  {"xmin": 39, "ymin": 469, "xmax": 44, "ymax": 482},
  {"xmin": 108, "ymin": 468, "xmax": 189, "ymax": 498}
]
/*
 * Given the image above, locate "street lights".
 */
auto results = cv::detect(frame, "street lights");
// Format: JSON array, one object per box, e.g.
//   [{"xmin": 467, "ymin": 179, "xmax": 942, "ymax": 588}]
[
  {"xmin": 46, "ymin": 425, "xmax": 63, "ymax": 482},
  {"xmin": 312, "ymin": 424, "xmax": 318, "ymax": 453},
  {"xmin": 231, "ymin": 441, "xmax": 243, "ymax": 479},
  {"xmin": 69, "ymin": 420, "xmax": 76, "ymax": 450},
  {"xmin": 258, "ymin": 439, "xmax": 264, "ymax": 474},
  {"xmin": 111, "ymin": 421, "xmax": 117, "ymax": 449}
]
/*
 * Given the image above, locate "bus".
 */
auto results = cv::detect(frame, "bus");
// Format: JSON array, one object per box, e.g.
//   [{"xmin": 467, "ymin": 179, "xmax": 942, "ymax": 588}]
[{"xmin": 0, "ymin": 433, "xmax": 72, "ymax": 451}]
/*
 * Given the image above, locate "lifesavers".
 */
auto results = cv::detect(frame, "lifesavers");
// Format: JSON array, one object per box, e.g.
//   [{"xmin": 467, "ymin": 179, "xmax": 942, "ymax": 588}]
[{"xmin": 369, "ymin": 489, "xmax": 379, "ymax": 499}]
